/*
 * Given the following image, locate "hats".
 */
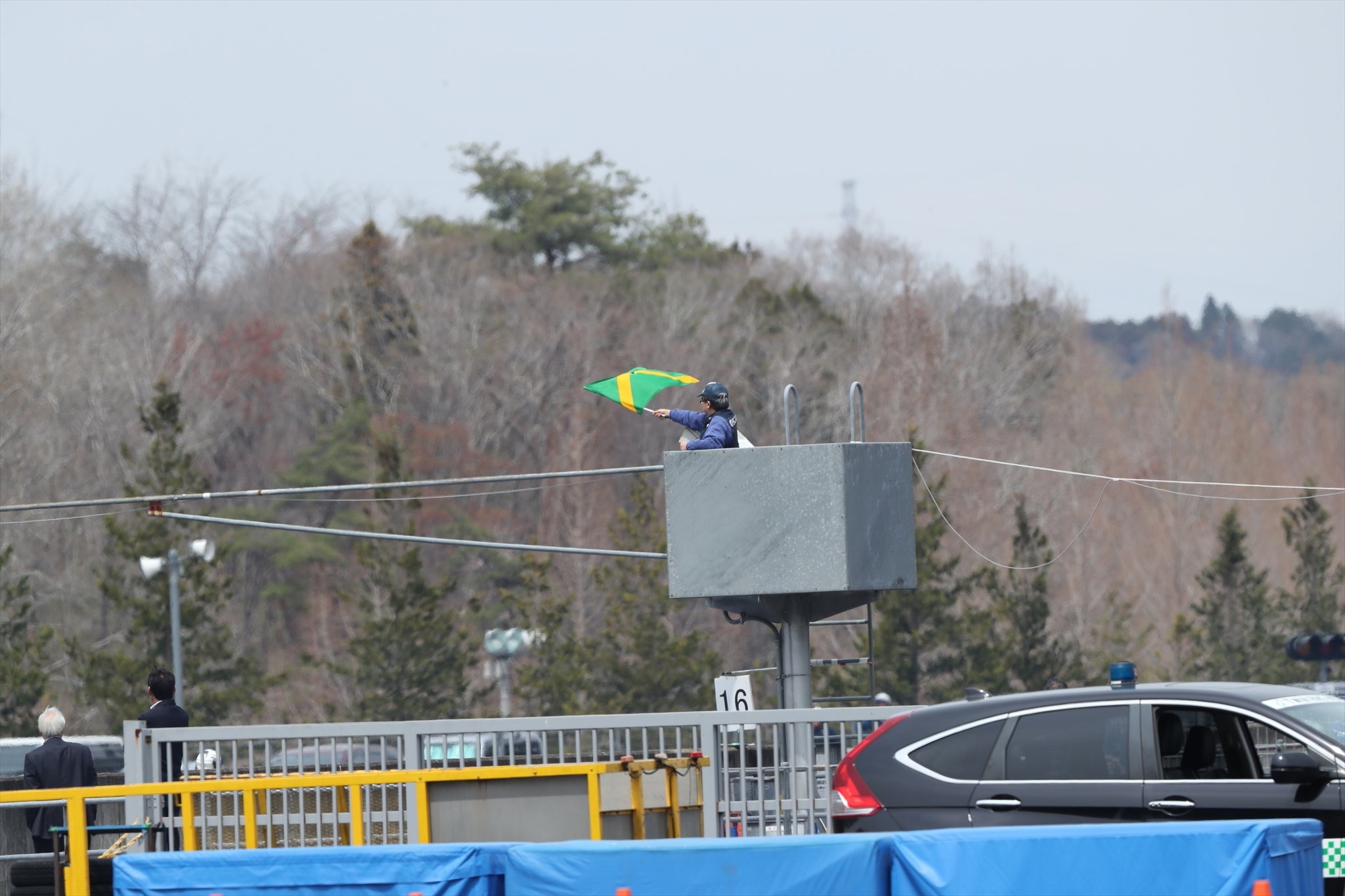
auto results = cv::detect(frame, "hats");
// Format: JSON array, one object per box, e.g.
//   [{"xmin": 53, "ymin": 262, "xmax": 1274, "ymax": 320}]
[{"xmin": 697, "ymin": 382, "xmax": 728, "ymax": 399}]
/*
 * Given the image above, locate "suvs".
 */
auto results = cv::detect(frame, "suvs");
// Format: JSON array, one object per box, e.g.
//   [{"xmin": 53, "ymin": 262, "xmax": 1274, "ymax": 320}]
[{"xmin": 830, "ymin": 661, "xmax": 1345, "ymax": 896}]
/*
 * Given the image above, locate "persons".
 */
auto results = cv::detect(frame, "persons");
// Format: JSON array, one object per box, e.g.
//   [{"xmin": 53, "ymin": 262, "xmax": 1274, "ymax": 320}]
[
  {"xmin": 138, "ymin": 666, "xmax": 188, "ymax": 852},
  {"xmin": 21, "ymin": 706, "xmax": 99, "ymax": 854},
  {"xmin": 196, "ymin": 749, "xmax": 223, "ymax": 770},
  {"xmin": 862, "ymin": 693, "xmax": 894, "ymax": 739},
  {"xmin": 813, "ymin": 706, "xmax": 841, "ymax": 743},
  {"xmin": 653, "ymin": 382, "xmax": 739, "ymax": 451}
]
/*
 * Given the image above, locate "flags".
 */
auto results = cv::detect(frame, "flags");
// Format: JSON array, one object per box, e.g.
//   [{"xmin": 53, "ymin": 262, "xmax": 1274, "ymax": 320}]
[{"xmin": 584, "ymin": 367, "xmax": 699, "ymax": 415}]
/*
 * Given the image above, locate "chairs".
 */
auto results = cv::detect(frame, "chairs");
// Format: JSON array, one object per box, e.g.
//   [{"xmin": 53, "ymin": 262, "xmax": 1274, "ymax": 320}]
[
  {"xmin": 1182, "ymin": 725, "xmax": 1228, "ymax": 779},
  {"xmin": 1158, "ymin": 713, "xmax": 1196, "ymax": 779}
]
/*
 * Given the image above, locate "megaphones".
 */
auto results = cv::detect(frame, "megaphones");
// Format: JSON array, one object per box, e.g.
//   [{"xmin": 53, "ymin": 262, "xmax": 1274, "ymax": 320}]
[
  {"xmin": 138, "ymin": 554, "xmax": 165, "ymax": 582},
  {"xmin": 187, "ymin": 538, "xmax": 216, "ymax": 562}
]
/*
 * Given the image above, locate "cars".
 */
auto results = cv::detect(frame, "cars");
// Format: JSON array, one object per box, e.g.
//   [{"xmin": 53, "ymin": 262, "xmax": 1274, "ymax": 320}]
[
  {"xmin": 423, "ymin": 731, "xmax": 543, "ymax": 761},
  {"xmin": 0, "ymin": 736, "xmax": 124, "ymax": 790},
  {"xmin": 264, "ymin": 743, "xmax": 404, "ymax": 767}
]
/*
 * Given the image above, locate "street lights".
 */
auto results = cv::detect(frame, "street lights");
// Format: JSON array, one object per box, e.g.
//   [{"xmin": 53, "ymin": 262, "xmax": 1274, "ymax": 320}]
[
  {"xmin": 138, "ymin": 539, "xmax": 217, "ymax": 711},
  {"xmin": 483, "ymin": 626, "xmax": 528, "ymax": 718}
]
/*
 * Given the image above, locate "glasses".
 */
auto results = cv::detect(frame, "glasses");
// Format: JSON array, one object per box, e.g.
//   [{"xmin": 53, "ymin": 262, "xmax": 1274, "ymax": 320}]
[{"xmin": 700, "ymin": 400, "xmax": 706, "ymax": 404}]
